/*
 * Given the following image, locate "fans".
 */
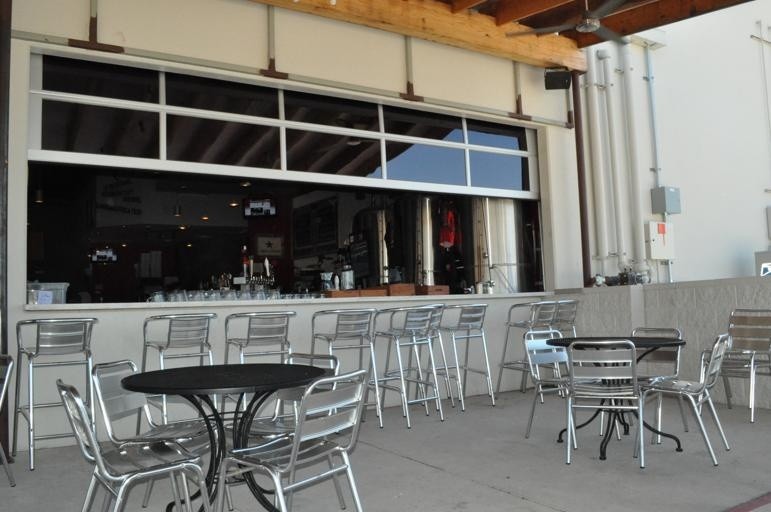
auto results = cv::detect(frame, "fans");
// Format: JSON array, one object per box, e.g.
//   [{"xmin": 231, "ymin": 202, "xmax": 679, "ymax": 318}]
[{"xmin": 504, "ymin": 0, "xmax": 635, "ymax": 46}]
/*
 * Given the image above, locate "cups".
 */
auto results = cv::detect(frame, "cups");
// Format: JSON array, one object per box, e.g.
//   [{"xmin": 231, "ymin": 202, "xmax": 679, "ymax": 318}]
[{"xmin": 147, "ymin": 289, "xmax": 282, "ymax": 303}]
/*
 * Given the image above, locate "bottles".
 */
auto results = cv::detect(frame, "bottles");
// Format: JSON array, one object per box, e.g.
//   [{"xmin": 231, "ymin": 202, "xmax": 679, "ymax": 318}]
[
  {"xmin": 621, "ymin": 265, "xmax": 629, "ymax": 285},
  {"xmin": 628, "ymin": 264, "xmax": 637, "ymax": 285}
]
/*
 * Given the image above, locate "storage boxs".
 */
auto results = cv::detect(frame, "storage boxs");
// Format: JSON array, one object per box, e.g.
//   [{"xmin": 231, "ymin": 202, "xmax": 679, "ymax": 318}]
[{"xmin": 326, "ymin": 280, "xmax": 450, "ymax": 296}]
